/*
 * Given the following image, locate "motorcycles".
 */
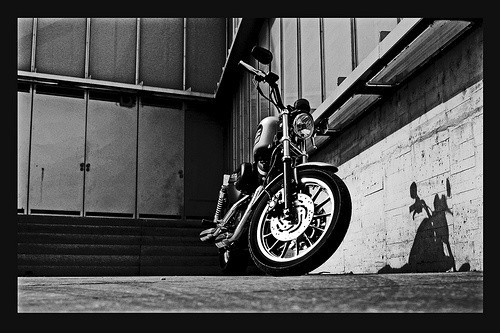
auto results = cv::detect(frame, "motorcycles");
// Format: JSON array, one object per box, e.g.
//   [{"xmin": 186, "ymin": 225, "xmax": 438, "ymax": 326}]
[{"xmin": 199, "ymin": 44, "xmax": 353, "ymax": 277}]
